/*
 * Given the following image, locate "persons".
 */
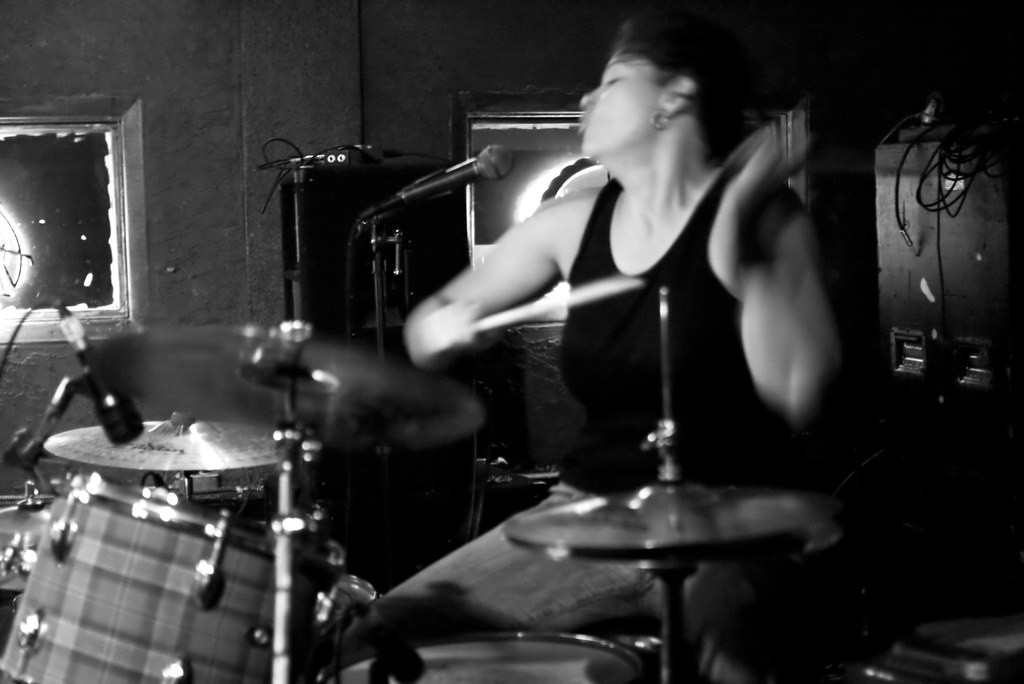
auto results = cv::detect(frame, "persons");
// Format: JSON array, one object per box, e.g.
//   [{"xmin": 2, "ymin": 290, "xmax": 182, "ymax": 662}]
[{"xmin": 331, "ymin": 2, "xmax": 837, "ymax": 668}]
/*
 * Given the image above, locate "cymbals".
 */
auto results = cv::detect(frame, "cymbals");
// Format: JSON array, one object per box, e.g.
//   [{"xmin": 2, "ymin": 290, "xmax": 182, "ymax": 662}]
[
  {"xmin": 39, "ymin": 412, "xmax": 322, "ymax": 475},
  {"xmin": 83, "ymin": 323, "xmax": 486, "ymax": 450},
  {"xmin": 501, "ymin": 482, "xmax": 842, "ymax": 559}
]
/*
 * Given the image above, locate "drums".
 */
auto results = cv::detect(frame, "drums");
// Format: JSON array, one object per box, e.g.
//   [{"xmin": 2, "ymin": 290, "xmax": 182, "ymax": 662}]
[
  {"xmin": 1, "ymin": 467, "xmax": 347, "ymax": 684},
  {"xmin": 313, "ymin": 629, "xmax": 645, "ymax": 683},
  {"xmin": 0, "ymin": 494, "xmax": 57, "ymax": 593}
]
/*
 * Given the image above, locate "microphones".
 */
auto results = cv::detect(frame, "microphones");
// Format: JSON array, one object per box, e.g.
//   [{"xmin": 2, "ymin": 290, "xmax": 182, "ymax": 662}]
[
  {"xmin": 356, "ymin": 145, "xmax": 515, "ymax": 220},
  {"xmin": 58, "ymin": 302, "xmax": 144, "ymax": 446},
  {"xmin": 350, "ymin": 602, "xmax": 425, "ymax": 684}
]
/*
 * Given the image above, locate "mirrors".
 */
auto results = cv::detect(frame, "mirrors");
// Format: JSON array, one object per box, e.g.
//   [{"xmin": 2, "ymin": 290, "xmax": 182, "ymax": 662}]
[
  {"xmin": 0, "ymin": 93, "xmax": 152, "ymax": 344},
  {"xmin": 446, "ymin": 85, "xmax": 814, "ymax": 334}
]
